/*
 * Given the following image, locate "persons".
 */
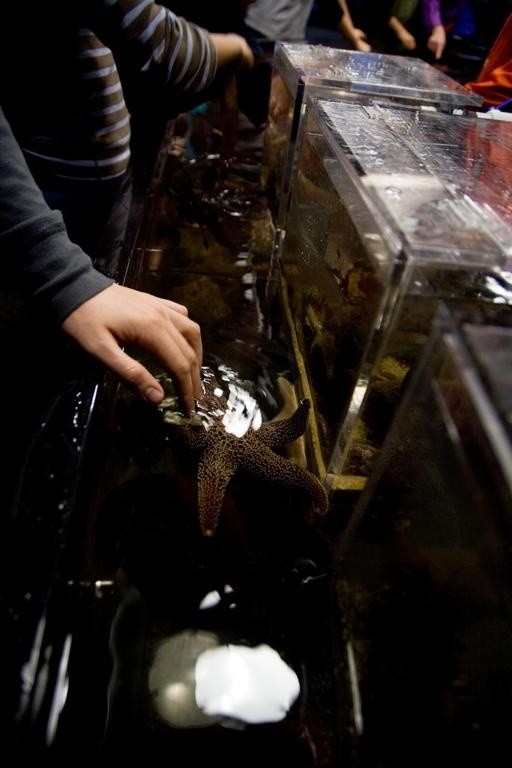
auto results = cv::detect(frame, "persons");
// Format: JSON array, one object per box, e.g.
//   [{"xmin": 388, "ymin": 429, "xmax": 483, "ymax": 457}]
[
  {"xmin": 379, "ymin": 1, "xmax": 422, "ymax": 51},
  {"xmin": 419, "ymin": 0, "xmax": 463, "ymax": 60},
  {"xmin": 0, "ymin": 1, "xmax": 255, "ymax": 278},
  {"xmin": 0, "ymin": 107, "xmax": 206, "ymax": 417},
  {"xmin": 242, "ymin": 1, "xmax": 372, "ymax": 87}
]
